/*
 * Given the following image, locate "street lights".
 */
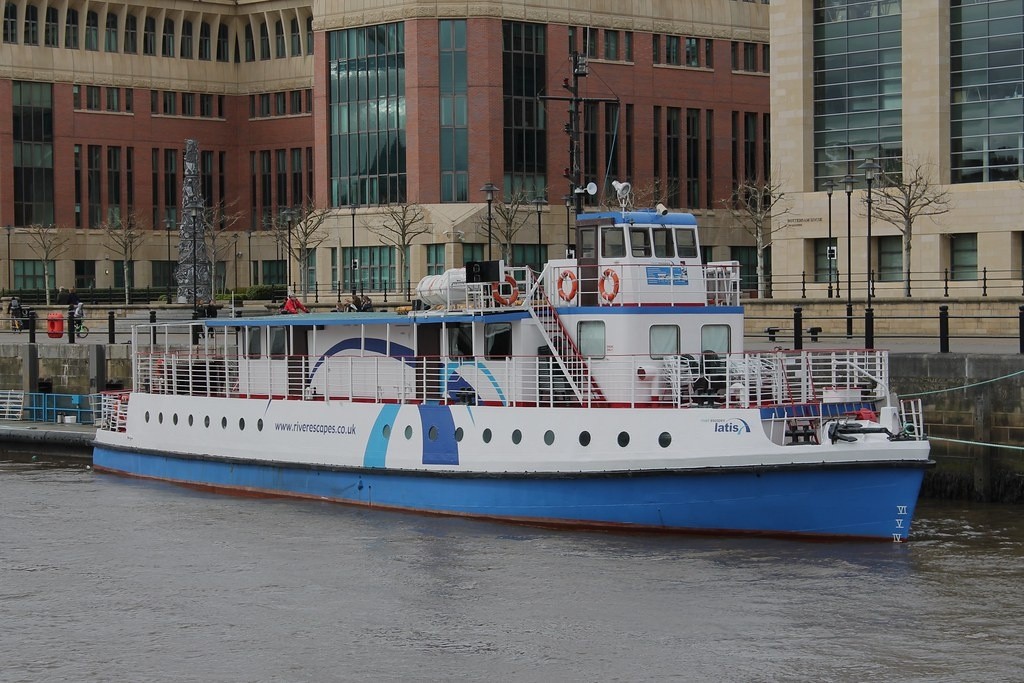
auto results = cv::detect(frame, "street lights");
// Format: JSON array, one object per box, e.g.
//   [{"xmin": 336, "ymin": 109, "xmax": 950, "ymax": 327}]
[
  {"xmin": 162, "ymin": 219, "xmax": 175, "ymax": 304},
  {"xmin": 821, "ymin": 181, "xmax": 839, "ymax": 298},
  {"xmin": 532, "ymin": 196, "xmax": 549, "ymax": 300},
  {"xmin": 561, "ymin": 194, "xmax": 574, "ymax": 254},
  {"xmin": 244, "ymin": 229, "xmax": 254, "ymax": 287},
  {"xmin": 347, "ymin": 203, "xmax": 360, "ymax": 295},
  {"xmin": 282, "ymin": 208, "xmax": 296, "ymax": 297},
  {"xmin": 185, "ymin": 204, "xmax": 205, "ymax": 344},
  {"xmin": 857, "ymin": 158, "xmax": 883, "ymax": 348},
  {"xmin": 4, "ymin": 225, "xmax": 14, "ymax": 290},
  {"xmin": 231, "ymin": 234, "xmax": 240, "ymax": 294},
  {"xmin": 837, "ymin": 175, "xmax": 860, "ymax": 339},
  {"xmin": 479, "ymin": 183, "xmax": 501, "ymax": 260}
]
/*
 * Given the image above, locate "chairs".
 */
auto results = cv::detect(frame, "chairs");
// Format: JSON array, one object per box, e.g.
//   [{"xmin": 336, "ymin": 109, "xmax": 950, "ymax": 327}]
[
  {"xmin": 661, "ymin": 354, "xmax": 776, "ymax": 401},
  {"xmin": 539, "ymin": 336, "xmax": 580, "ymax": 405}
]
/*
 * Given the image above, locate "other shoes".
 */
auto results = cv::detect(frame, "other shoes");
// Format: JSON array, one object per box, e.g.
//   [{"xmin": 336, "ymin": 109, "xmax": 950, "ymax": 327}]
[
  {"xmin": 74, "ymin": 330, "xmax": 80, "ymax": 334},
  {"xmin": 202, "ymin": 334, "xmax": 205, "ymax": 338},
  {"xmin": 198, "ymin": 336, "xmax": 201, "ymax": 338},
  {"xmin": 211, "ymin": 334, "xmax": 214, "ymax": 338}
]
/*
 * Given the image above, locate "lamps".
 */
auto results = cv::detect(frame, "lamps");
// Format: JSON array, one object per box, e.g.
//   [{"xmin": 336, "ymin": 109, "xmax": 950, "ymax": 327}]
[{"xmin": 612, "ymin": 180, "xmax": 630, "ymax": 198}]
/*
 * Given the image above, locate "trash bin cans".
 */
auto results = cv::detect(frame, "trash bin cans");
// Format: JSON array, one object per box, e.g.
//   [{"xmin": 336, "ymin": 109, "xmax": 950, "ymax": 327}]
[{"xmin": 11, "ymin": 307, "xmax": 35, "ymax": 331}]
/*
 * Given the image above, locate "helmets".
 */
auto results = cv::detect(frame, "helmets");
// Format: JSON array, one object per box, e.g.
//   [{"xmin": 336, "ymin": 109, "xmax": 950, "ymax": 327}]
[{"xmin": 290, "ymin": 294, "xmax": 295, "ymax": 297}]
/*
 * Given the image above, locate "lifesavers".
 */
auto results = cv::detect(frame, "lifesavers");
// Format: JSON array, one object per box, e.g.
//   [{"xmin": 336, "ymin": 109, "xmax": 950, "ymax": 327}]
[
  {"xmin": 492, "ymin": 275, "xmax": 518, "ymax": 304},
  {"xmin": 558, "ymin": 269, "xmax": 577, "ymax": 301},
  {"xmin": 156, "ymin": 358, "xmax": 164, "ymax": 380},
  {"xmin": 599, "ymin": 269, "xmax": 619, "ymax": 302}
]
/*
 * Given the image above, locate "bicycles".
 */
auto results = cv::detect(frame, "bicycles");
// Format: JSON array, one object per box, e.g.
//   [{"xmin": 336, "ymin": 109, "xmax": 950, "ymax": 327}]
[
  {"xmin": 7, "ymin": 313, "xmax": 22, "ymax": 334},
  {"xmin": 74, "ymin": 317, "xmax": 89, "ymax": 338}
]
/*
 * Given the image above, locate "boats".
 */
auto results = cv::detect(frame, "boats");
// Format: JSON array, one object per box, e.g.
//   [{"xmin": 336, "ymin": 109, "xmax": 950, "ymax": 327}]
[
  {"xmin": 91, "ymin": 48, "xmax": 938, "ymax": 542},
  {"xmin": 415, "ymin": 269, "xmax": 481, "ymax": 306}
]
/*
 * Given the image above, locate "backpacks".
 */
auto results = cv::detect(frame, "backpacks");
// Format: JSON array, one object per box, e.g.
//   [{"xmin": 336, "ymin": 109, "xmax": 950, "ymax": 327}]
[{"xmin": 12, "ymin": 300, "xmax": 18, "ymax": 306}]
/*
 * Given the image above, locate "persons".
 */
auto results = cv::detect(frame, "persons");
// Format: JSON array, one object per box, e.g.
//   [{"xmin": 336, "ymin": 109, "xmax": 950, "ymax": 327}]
[
  {"xmin": 74, "ymin": 302, "xmax": 86, "ymax": 337},
  {"xmin": 6, "ymin": 297, "xmax": 23, "ymax": 335},
  {"xmin": 278, "ymin": 293, "xmax": 310, "ymax": 314},
  {"xmin": 336, "ymin": 295, "xmax": 373, "ymax": 312}
]
